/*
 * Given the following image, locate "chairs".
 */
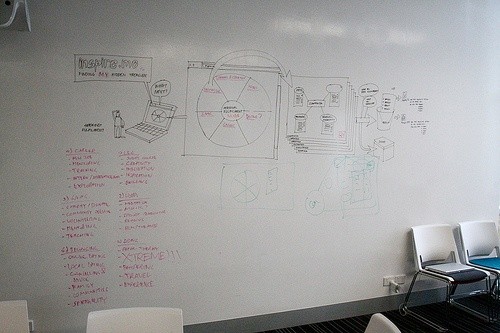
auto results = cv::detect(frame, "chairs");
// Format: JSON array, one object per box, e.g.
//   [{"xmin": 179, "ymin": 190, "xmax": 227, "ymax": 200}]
[
  {"xmin": 86, "ymin": 307, "xmax": 183, "ymax": 333},
  {"xmin": 0, "ymin": 300, "xmax": 29, "ymax": 333},
  {"xmin": 456, "ymin": 220, "xmax": 500, "ymax": 323},
  {"xmin": 403, "ymin": 224, "xmax": 495, "ymax": 333},
  {"xmin": 364, "ymin": 313, "xmax": 401, "ymax": 333}
]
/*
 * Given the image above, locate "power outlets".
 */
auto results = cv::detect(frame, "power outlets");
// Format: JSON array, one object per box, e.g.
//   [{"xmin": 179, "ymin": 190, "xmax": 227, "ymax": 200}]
[{"xmin": 383, "ymin": 274, "xmax": 405, "ymax": 286}]
